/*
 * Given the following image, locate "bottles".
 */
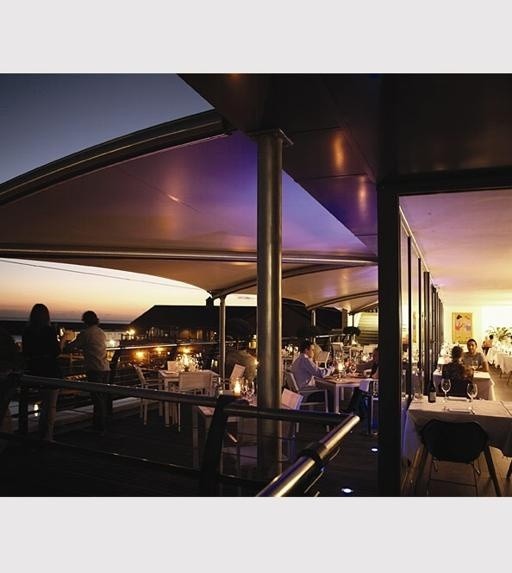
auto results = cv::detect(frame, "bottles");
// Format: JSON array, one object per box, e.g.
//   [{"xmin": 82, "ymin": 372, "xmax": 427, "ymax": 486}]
[{"xmin": 428, "ymin": 372, "xmax": 437, "ymax": 403}]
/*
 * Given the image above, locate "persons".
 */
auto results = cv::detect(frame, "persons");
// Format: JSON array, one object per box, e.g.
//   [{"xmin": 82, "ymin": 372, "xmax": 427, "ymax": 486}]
[
  {"xmin": 483, "ymin": 335, "xmax": 490, "ymax": 344},
  {"xmin": 463, "ymin": 314, "xmax": 472, "ymax": 332},
  {"xmin": 485, "ymin": 334, "xmax": 494, "ymax": 356},
  {"xmin": 292, "ymin": 340, "xmax": 337, "ymax": 429},
  {"xmin": 339, "ymin": 346, "xmax": 379, "ymax": 420},
  {"xmin": 1, "ymin": 327, "xmax": 23, "ymax": 453},
  {"xmin": 65, "ymin": 310, "xmax": 110, "ymax": 438},
  {"xmin": 458, "ymin": 338, "xmax": 489, "ymax": 373},
  {"xmin": 435, "ymin": 345, "xmax": 474, "ymax": 396},
  {"xmin": 23, "ymin": 303, "xmax": 72, "ymax": 448},
  {"xmin": 454, "ymin": 314, "xmax": 464, "ymax": 331}
]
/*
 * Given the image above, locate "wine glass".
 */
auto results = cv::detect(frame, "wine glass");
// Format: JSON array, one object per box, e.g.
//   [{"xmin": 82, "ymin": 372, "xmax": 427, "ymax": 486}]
[
  {"xmin": 466, "ymin": 383, "xmax": 478, "ymax": 410},
  {"xmin": 441, "ymin": 378, "xmax": 452, "ymax": 404}
]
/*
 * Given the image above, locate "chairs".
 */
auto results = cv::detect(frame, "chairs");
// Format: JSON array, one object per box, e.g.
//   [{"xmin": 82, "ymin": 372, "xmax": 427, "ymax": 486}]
[{"xmin": 131, "ymin": 339, "xmax": 378, "ymax": 461}]
